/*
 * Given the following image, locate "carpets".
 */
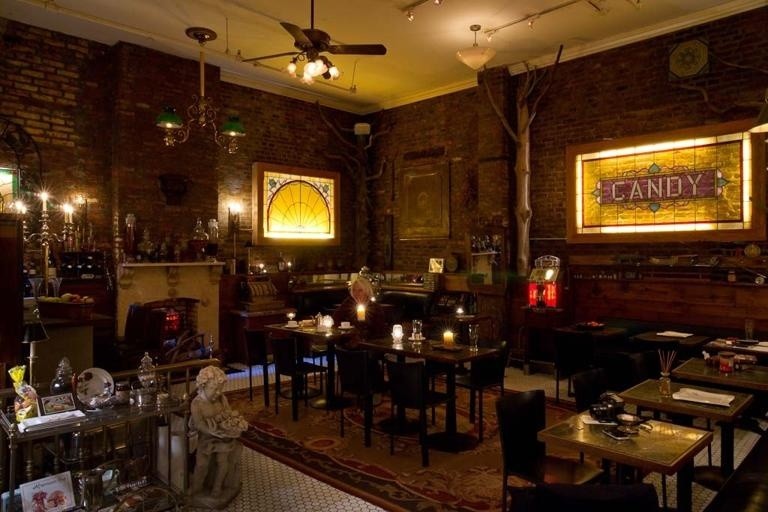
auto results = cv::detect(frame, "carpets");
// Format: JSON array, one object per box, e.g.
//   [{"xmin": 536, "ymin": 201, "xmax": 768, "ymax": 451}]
[{"xmin": 225, "ymin": 377, "xmax": 589, "ymax": 512}]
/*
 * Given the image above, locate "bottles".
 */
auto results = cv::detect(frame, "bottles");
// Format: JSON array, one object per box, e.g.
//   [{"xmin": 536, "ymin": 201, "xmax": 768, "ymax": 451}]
[
  {"xmin": 727, "ymin": 269, "xmax": 735, "ymax": 282},
  {"xmin": 115, "ymin": 381, "xmax": 130, "ymax": 405},
  {"xmin": 276, "ymin": 252, "xmax": 287, "ymax": 272},
  {"xmin": 571, "ymin": 273, "xmax": 583, "ymax": 279},
  {"xmin": 658, "ymin": 374, "xmax": 671, "ymax": 397},
  {"xmin": 50, "ymin": 357, "xmax": 75, "ymax": 396},
  {"xmin": 25, "ymin": 247, "xmax": 103, "ymax": 278},
  {"xmin": 706, "ymin": 352, "xmax": 735, "ymax": 372},
  {"xmin": 123, "ymin": 212, "xmax": 219, "ymax": 263}
]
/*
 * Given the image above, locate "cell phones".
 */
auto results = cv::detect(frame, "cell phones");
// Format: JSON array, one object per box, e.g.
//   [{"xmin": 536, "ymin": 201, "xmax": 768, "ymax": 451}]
[{"xmin": 601, "ymin": 428, "xmax": 630, "ymax": 440}]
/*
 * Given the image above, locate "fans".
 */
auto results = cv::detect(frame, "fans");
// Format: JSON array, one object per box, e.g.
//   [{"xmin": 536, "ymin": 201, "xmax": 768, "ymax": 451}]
[{"xmin": 241, "ymin": 0, "xmax": 386, "ymax": 78}]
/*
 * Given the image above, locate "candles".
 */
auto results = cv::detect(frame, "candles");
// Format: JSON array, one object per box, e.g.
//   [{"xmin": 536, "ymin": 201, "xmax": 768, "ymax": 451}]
[
  {"xmin": 64, "ymin": 204, "xmax": 69, "ymax": 222},
  {"xmin": 357, "ymin": 305, "xmax": 366, "ymax": 320},
  {"xmin": 41, "ymin": 192, "xmax": 49, "ymax": 211}
]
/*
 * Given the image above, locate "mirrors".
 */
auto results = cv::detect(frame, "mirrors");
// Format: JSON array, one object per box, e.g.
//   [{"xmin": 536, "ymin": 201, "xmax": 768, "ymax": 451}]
[{"xmin": 0, "ymin": 167, "xmax": 22, "ymax": 214}]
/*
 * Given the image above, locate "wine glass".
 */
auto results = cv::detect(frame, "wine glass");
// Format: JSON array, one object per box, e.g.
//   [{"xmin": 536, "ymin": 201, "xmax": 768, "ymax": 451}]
[{"xmin": 28, "ymin": 278, "xmax": 62, "ymax": 303}]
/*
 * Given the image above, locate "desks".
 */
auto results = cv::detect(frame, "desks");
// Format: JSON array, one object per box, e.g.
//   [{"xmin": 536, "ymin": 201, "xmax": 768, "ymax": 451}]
[
  {"xmin": 262, "ymin": 320, "xmax": 497, "ymax": 455},
  {"xmin": 554, "ymin": 313, "xmax": 768, "ymax": 429},
  {"xmin": 537, "ymin": 405, "xmax": 715, "ymax": 511},
  {"xmin": 1, "ymin": 388, "xmax": 192, "ymax": 512},
  {"xmin": 672, "ymin": 356, "xmax": 767, "ymax": 493},
  {"xmin": 617, "ymin": 378, "xmax": 753, "ymax": 511}
]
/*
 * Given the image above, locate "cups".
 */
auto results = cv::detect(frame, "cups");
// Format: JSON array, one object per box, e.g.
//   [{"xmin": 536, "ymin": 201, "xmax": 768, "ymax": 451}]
[
  {"xmin": 287, "ymin": 321, "xmax": 297, "ymax": 326},
  {"xmin": 468, "ymin": 323, "xmax": 478, "ymax": 351},
  {"xmin": 614, "ymin": 413, "xmax": 652, "ymax": 433},
  {"xmin": 341, "ymin": 322, "xmax": 351, "ymax": 327},
  {"xmin": 744, "ymin": 319, "xmax": 752, "ymax": 338},
  {"xmin": 412, "ymin": 320, "xmax": 423, "ymax": 339}
]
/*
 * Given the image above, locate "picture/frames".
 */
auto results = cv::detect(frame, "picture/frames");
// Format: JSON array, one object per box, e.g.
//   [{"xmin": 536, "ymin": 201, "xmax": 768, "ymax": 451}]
[{"xmin": 18, "ymin": 469, "xmax": 76, "ymax": 512}]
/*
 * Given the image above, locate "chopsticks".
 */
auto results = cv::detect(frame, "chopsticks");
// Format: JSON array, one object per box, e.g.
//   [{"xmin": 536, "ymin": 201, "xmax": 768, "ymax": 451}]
[{"xmin": 658, "ymin": 349, "xmax": 677, "ymax": 373}]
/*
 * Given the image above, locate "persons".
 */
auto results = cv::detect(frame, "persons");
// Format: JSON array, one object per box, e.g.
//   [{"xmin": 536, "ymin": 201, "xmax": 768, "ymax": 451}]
[
  {"xmin": 186, "ymin": 364, "xmax": 247, "ymax": 498},
  {"xmin": 330, "ymin": 274, "xmax": 388, "ymax": 390}
]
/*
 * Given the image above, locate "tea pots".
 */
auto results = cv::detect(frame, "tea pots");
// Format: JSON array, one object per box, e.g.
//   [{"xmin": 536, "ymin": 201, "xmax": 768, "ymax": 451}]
[
  {"xmin": 130, "ymin": 377, "xmax": 157, "ymax": 408},
  {"xmin": 72, "ymin": 467, "xmax": 117, "ymax": 509},
  {"xmin": 309, "ymin": 312, "xmax": 324, "ymax": 327}
]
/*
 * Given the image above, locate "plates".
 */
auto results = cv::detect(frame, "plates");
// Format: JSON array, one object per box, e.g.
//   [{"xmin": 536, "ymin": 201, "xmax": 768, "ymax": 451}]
[
  {"xmin": 338, "ymin": 326, "xmax": 355, "ymax": 329},
  {"xmin": 673, "ymin": 387, "xmax": 735, "ymax": 407},
  {"xmin": 285, "ymin": 324, "xmax": 299, "ymax": 329},
  {"xmin": 74, "ymin": 368, "xmax": 116, "ymax": 405},
  {"xmin": 574, "ymin": 321, "xmax": 608, "ymax": 329},
  {"xmin": 744, "ymin": 244, "xmax": 762, "ymax": 258}
]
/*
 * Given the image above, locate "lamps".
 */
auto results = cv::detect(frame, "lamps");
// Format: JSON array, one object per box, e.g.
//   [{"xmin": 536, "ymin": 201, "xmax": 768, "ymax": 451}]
[
  {"xmin": 154, "ymin": 26, "xmax": 245, "ymax": 155},
  {"xmin": 284, "ymin": 51, "xmax": 340, "ymax": 86},
  {"xmin": 456, "ymin": 25, "xmax": 497, "ymax": 72}
]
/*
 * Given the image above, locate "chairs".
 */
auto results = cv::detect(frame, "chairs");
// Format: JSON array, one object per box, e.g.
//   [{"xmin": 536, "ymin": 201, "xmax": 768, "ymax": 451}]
[
  {"xmin": 452, "ymin": 350, "xmax": 508, "ymax": 444},
  {"xmin": 384, "ymin": 358, "xmax": 451, "ymax": 469},
  {"xmin": 243, "ymin": 328, "xmax": 273, "ymax": 408},
  {"xmin": 268, "ymin": 336, "xmax": 330, "ymax": 423},
  {"xmin": 495, "ymin": 389, "xmax": 660, "ymax": 510},
  {"xmin": 332, "ymin": 347, "xmax": 390, "ymax": 448}
]
